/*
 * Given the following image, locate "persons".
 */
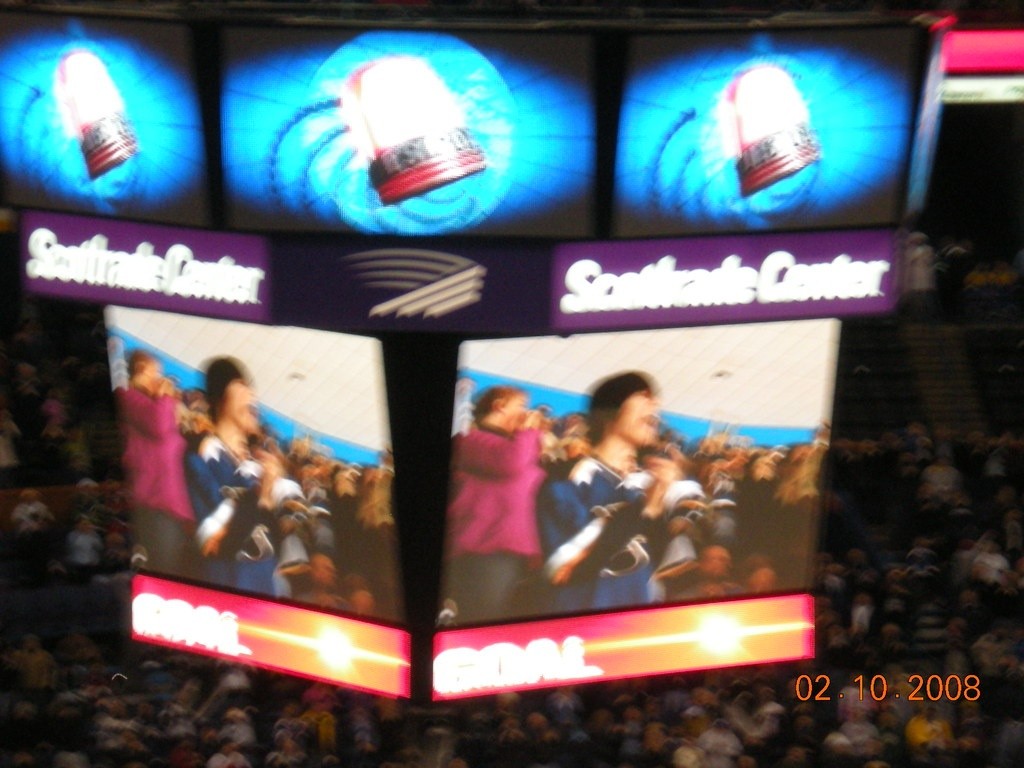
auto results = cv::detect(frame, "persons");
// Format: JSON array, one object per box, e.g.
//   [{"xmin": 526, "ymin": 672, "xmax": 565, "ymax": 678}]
[
  {"xmin": 444, "ymin": 374, "xmax": 829, "ymax": 621},
  {"xmin": 1, "ymin": 225, "xmax": 1024, "ymax": 768},
  {"xmin": 112, "ymin": 349, "xmax": 392, "ymax": 615}
]
